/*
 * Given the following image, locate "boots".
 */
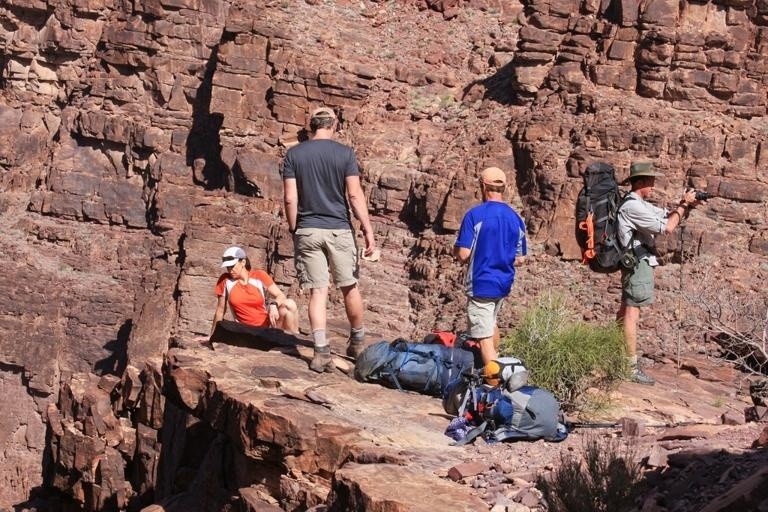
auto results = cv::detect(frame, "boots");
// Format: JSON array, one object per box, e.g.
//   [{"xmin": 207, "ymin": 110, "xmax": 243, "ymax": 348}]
[
  {"xmin": 346, "ymin": 337, "xmax": 366, "ymax": 359},
  {"xmin": 309, "ymin": 345, "xmax": 338, "ymax": 374}
]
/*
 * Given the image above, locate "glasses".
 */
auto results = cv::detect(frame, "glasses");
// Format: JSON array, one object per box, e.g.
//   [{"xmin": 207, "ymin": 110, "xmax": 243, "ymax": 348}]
[{"xmin": 221, "ymin": 256, "xmax": 239, "ymax": 262}]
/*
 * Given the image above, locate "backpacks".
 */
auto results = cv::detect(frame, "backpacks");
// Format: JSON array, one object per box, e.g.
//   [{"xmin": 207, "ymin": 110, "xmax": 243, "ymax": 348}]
[
  {"xmin": 575, "ymin": 162, "xmax": 660, "ymax": 274},
  {"xmin": 443, "ymin": 374, "xmax": 574, "ymax": 448},
  {"xmin": 354, "ymin": 338, "xmax": 475, "ymax": 401}
]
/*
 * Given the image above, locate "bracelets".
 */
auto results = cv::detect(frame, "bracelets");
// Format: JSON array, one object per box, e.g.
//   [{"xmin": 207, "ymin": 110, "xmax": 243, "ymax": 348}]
[
  {"xmin": 678, "ymin": 204, "xmax": 687, "ymax": 210},
  {"xmin": 289, "ymin": 226, "xmax": 300, "ymax": 235},
  {"xmin": 269, "ymin": 301, "xmax": 278, "ymax": 307}
]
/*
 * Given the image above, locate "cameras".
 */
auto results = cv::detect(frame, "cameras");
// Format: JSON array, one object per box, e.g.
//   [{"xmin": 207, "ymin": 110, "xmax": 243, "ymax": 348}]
[{"xmin": 685, "ymin": 187, "xmax": 710, "ymax": 209}]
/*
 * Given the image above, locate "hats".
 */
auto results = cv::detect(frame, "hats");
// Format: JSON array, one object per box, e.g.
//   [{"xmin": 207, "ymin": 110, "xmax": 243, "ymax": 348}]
[
  {"xmin": 218, "ymin": 247, "xmax": 247, "ymax": 270},
  {"xmin": 621, "ymin": 161, "xmax": 666, "ymax": 185},
  {"xmin": 311, "ymin": 108, "xmax": 336, "ymax": 121},
  {"xmin": 479, "ymin": 167, "xmax": 507, "ymax": 188}
]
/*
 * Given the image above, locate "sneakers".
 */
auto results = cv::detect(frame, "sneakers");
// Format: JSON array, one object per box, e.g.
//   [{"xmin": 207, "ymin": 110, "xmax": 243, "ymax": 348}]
[{"xmin": 625, "ymin": 363, "xmax": 655, "ymax": 384}]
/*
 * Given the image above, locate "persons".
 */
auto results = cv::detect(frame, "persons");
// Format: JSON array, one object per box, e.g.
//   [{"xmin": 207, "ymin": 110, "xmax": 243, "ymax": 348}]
[
  {"xmin": 282, "ymin": 107, "xmax": 380, "ymax": 372},
  {"xmin": 189, "ymin": 246, "xmax": 301, "ymax": 342},
  {"xmin": 613, "ymin": 160, "xmax": 702, "ymax": 384},
  {"xmin": 453, "ymin": 166, "xmax": 527, "ymax": 385}
]
